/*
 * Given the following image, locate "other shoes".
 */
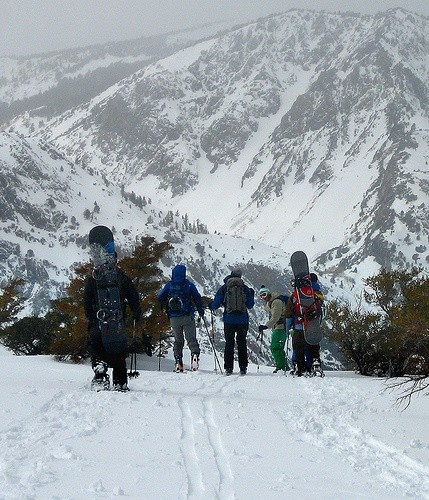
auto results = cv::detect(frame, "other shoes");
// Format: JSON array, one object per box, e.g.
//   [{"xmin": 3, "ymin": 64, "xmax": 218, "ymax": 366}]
[
  {"xmin": 240, "ymin": 366, "xmax": 247, "ymax": 375},
  {"xmin": 226, "ymin": 368, "xmax": 233, "ymax": 376},
  {"xmin": 191, "ymin": 354, "xmax": 199, "ymax": 371},
  {"xmin": 114, "ymin": 384, "xmax": 130, "ymax": 393},
  {"xmin": 272, "ymin": 368, "xmax": 290, "ymax": 373},
  {"xmin": 175, "ymin": 363, "xmax": 184, "ymax": 373}
]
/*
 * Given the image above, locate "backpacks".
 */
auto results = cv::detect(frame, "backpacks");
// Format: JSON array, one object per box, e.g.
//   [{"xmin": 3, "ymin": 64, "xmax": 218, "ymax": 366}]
[
  {"xmin": 222, "ymin": 277, "xmax": 247, "ymax": 314},
  {"xmin": 164, "ymin": 279, "xmax": 193, "ymax": 314},
  {"xmin": 268, "ymin": 295, "xmax": 289, "ymax": 329}
]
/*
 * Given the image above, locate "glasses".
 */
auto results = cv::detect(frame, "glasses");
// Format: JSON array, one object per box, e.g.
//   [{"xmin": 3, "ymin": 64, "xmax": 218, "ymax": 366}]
[{"xmin": 258, "ymin": 291, "xmax": 269, "ymax": 300}]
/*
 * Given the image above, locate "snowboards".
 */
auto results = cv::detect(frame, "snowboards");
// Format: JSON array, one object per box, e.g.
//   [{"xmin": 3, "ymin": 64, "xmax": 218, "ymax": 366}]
[
  {"xmin": 88, "ymin": 225, "xmax": 128, "ymax": 352},
  {"xmin": 290, "ymin": 250, "xmax": 323, "ymax": 345}
]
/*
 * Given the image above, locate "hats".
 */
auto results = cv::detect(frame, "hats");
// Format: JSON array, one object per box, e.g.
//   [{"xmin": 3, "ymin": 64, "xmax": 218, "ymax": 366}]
[
  {"xmin": 309, "ymin": 273, "xmax": 319, "ymax": 284},
  {"xmin": 231, "ymin": 269, "xmax": 243, "ymax": 278},
  {"xmin": 257, "ymin": 288, "xmax": 271, "ymax": 296}
]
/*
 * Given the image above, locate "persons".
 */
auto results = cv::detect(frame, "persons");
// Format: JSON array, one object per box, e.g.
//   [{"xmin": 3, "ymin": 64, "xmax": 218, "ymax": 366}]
[
  {"xmin": 259, "ymin": 285, "xmax": 290, "ymax": 373},
  {"xmin": 208, "ymin": 267, "xmax": 254, "ymax": 376},
  {"xmin": 285, "ymin": 273, "xmax": 324, "ymax": 377},
  {"xmin": 157, "ymin": 265, "xmax": 205, "ymax": 373},
  {"xmin": 84, "ymin": 252, "xmax": 141, "ymax": 393}
]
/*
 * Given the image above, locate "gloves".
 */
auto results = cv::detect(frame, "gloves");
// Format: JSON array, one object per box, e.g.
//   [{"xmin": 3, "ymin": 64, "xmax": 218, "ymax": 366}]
[
  {"xmin": 259, "ymin": 325, "xmax": 268, "ymax": 332},
  {"xmin": 131, "ymin": 306, "xmax": 141, "ymax": 323},
  {"xmin": 198, "ymin": 310, "xmax": 205, "ymax": 317},
  {"xmin": 208, "ymin": 301, "xmax": 214, "ymax": 310}
]
